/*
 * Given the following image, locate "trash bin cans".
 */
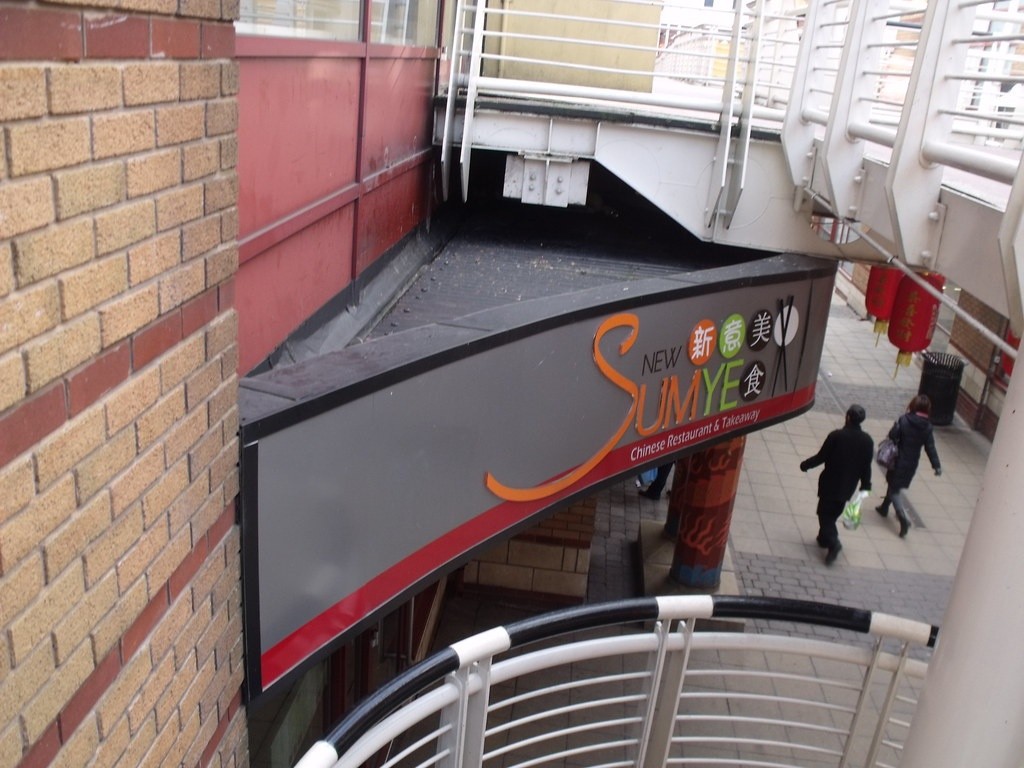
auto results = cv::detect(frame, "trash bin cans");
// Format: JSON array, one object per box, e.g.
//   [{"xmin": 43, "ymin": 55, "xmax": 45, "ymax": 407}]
[{"xmin": 917, "ymin": 351, "xmax": 970, "ymax": 427}]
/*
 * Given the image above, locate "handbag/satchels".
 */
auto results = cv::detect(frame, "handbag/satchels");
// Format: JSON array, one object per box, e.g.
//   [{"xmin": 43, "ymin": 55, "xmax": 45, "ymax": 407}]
[
  {"xmin": 843, "ymin": 491, "xmax": 870, "ymax": 530},
  {"xmin": 876, "ymin": 420, "xmax": 900, "ymax": 470}
]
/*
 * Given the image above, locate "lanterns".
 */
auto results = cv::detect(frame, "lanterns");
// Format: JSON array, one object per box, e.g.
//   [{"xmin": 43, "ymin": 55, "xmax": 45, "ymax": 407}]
[
  {"xmin": 888, "ymin": 274, "xmax": 942, "ymax": 378},
  {"xmin": 1002, "ymin": 329, "xmax": 1020, "ymax": 377},
  {"xmin": 866, "ymin": 267, "xmax": 903, "ymax": 348}
]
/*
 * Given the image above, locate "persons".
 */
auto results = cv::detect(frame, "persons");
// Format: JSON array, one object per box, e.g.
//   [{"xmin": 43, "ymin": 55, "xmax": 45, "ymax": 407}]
[
  {"xmin": 875, "ymin": 394, "xmax": 941, "ymax": 537},
  {"xmin": 638, "ymin": 461, "xmax": 676, "ymax": 501},
  {"xmin": 800, "ymin": 404, "xmax": 874, "ymax": 565}
]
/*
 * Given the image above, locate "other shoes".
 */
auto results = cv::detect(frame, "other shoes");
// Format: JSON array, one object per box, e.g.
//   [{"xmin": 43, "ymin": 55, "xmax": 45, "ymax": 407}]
[
  {"xmin": 639, "ymin": 490, "xmax": 660, "ymax": 500},
  {"xmin": 825, "ymin": 542, "xmax": 842, "ymax": 566},
  {"xmin": 817, "ymin": 535, "xmax": 827, "ymax": 548}
]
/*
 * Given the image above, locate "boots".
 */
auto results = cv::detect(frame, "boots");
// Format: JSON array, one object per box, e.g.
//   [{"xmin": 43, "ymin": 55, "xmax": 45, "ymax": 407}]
[
  {"xmin": 896, "ymin": 509, "xmax": 911, "ymax": 537},
  {"xmin": 875, "ymin": 496, "xmax": 892, "ymax": 517}
]
